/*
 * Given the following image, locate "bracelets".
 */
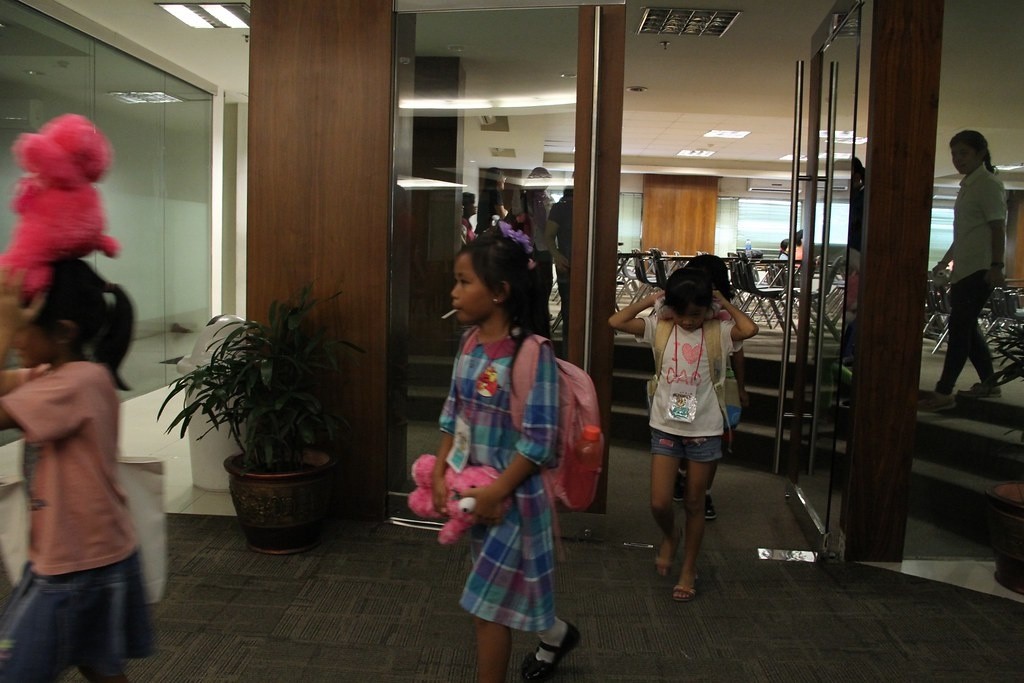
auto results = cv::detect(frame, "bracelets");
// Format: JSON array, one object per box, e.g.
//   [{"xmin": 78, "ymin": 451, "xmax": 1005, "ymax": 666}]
[{"xmin": 938, "ymin": 262, "xmax": 948, "ymax": 266}]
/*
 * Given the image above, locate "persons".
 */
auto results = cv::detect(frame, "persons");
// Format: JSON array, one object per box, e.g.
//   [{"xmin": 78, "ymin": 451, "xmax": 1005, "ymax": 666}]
[
  {"xmin": 462, "ymin": 167, "xmax": 570, "ymax": 362},
  {"xmin": 430, "ymin": 221, "xmax": 580, "ymax": 683},
  {"xmin": 918, "ymin": 131, "xmax": 1008, "ymax": 409},
  {"xmin": 607, "ymin": 156, "xmax": 866, "ymax": 601},
  {"xmin": 0, "ymin": 259, "xmax": 156, "ymax": 683}
]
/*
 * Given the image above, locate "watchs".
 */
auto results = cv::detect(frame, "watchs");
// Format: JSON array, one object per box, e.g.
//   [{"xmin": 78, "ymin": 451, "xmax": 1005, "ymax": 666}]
[{"xmin": 991, "ymin": 261, "xmax": 1005, "ymax": 268}]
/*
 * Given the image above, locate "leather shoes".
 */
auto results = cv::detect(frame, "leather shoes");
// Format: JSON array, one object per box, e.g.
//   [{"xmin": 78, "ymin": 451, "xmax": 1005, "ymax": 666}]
[{"xmin": 521, "ymin": 622, "xmax": 580, "ymax": 683}]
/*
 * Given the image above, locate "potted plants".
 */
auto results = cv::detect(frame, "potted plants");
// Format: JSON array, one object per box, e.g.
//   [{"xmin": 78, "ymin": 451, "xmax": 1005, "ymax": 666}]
[
  {"xmin": 982, "ymin": 283, "xmax": 1024, "ymax": 595},
  {"xmin": 157, "ymin": 276, "xmax": 368, "ymax": 554}
]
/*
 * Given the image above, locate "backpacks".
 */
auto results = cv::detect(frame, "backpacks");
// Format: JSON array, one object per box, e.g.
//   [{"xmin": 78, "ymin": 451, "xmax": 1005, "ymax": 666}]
[
  {"xmin": 647, "ymin": 320, "xmax": 742, "ymax": 454},
  {"xmin": 462, "ymin": 328, "xmax": 604, "ymax": 511}
]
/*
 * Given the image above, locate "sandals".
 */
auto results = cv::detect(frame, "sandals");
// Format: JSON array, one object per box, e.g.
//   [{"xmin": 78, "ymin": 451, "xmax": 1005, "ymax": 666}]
[
  {"xmin": 671, "ymin": 584, "xmax": 696, "ymax": 600},
  {"xmin": 653, "ymin": 526, "xmax": 682, "ymax": 578}
]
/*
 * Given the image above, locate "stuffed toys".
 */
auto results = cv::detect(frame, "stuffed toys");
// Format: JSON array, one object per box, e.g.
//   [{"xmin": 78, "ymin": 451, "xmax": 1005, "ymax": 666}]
[
  {"xmin": 0, "ymin": 113, "xmax": 119, "ymax": 300},
  {"xmin": 409, "ymin": 455, "xmax": 511, "ymax": 543},
  {"xmin": 931, "ymin": 270, "xmax": 950, "ymax": 289}
]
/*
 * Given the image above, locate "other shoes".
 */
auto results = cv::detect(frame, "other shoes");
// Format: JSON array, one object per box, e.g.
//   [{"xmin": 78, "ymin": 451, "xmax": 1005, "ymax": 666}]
[
  {"xmin": 673, "ymin": 470, "xmax": 687, "ymax": 502},
  {"xmin": 916, "ymin": 393, "xmax": 957, "ymax": 413},
  {"xmin": 957, "ymin": 383, "xmax": 1002, "ymax": 398},
  {"xmin": 704, "ymin": 493, "xmax": 718, "ymax": 519}
]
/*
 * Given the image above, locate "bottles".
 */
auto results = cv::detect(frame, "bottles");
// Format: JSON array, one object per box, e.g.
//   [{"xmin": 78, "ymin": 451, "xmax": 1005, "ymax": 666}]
[{"xmin": 746, "ymin": 238, "xmax": 751, "ymax": 258}]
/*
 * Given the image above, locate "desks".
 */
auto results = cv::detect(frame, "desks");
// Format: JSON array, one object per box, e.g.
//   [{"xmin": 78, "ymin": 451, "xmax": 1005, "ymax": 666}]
[
  {"xmin": 749, "ymin": 256, "xmax": 803, "ymax": 265},
  {"xmin": 618, "ymin": 251, "xmax": 636, "ymax": 258},
  {"xmin": 663, "ymin": 254, "xmax": 739, "ymax": 261}
]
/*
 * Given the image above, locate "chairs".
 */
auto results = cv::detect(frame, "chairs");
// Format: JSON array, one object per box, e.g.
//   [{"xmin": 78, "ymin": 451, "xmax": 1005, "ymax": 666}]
[
  {"xmin": 552, "ymin": 247, "xmax": 847, "ymax": 343},
  {"xmin": 922, "ymin": 276, "xmax": 1024, "ymax": 355}
]
